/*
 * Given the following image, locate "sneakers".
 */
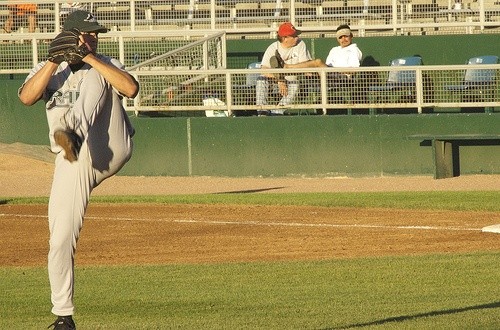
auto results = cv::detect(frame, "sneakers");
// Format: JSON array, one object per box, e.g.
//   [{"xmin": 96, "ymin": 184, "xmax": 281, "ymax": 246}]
[
  {"xmin": 54, "ymin": 130, "xmax": 82, "ymax": 162},
  {"xmin": 47, "ymin": 316, "xmax": 75, "ymax": 330}
]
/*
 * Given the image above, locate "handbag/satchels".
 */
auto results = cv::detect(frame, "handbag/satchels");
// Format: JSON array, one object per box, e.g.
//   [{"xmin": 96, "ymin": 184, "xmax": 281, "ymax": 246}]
[{"xmin": 202, "ymin": 97, "xmax": 235, "ymax": 118}]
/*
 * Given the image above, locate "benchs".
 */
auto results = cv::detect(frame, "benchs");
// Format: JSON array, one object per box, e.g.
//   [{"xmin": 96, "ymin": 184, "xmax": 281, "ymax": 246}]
[{"xmin": 407, "ymin": 132, "xmax": 500, "ymax": 180}]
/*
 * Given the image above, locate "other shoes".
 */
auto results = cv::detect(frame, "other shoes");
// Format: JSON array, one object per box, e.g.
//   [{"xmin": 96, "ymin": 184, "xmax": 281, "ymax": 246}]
[
  {"xmin": 258, "ymin": 110, "xmax": 268, "ymax": 116},
  {"xmin": 270, "ymin": 52, "xmax": 284, "ymax": 69}
]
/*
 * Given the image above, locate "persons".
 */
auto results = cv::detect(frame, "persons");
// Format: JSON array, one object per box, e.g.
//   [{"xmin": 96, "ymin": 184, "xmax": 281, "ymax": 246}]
[
  {"xmin": 270, "ymin": 25, "xmax": 363, "ymax": 75},
  {"xmin": 61, "ymin": 3, "xmax": 85, "ymax": 13},
  {"xmin": 18, "ymin": 11, "xmax": 139, "ymax": 330},
  {"xmin": 5, "ymin": 5, "xmax": 37, "ymax": 33},
  {"xmin": 256, "ymin": 23, "xmax": 310, "ymax": 115}
]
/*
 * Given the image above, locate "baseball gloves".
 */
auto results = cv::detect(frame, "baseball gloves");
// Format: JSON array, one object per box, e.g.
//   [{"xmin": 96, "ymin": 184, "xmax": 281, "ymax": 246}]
[{"xmin": 46, "ymin": 30, "xmax": 91, "ymax": 65}]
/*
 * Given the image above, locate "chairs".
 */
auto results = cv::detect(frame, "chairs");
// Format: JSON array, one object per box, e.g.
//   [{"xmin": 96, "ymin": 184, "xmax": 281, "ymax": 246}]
[
  {"xmin": 244, "ymin": 55, "xmax": 500, "ymax": 111},
  {"xmin": 0, "ymin": 0, "xmax": 500, "ymax": 38}
]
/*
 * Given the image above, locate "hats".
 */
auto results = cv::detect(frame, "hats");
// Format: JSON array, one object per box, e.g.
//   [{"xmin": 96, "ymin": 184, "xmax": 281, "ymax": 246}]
[
  {"xmin": 336, "ymin": 29, "xmax": 352, "ymax": 39},
  {"xmin": 278, "ymin": 23, "xmax": 301, "ymax": 37},
  {"xmin": 64, "ymin": 10, "xmax": 107, "ymax": 34}
]
